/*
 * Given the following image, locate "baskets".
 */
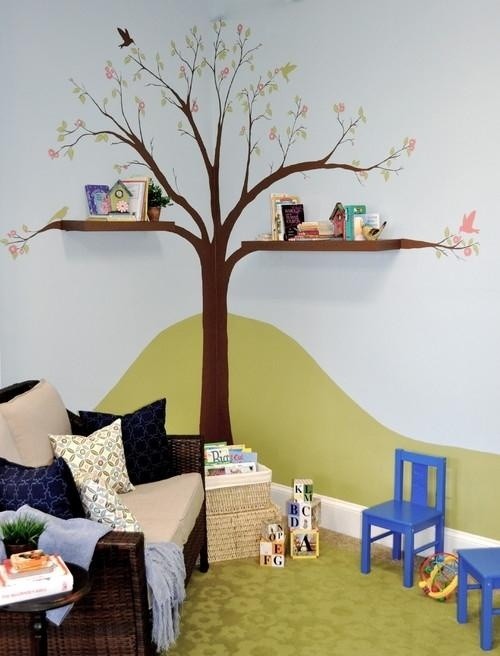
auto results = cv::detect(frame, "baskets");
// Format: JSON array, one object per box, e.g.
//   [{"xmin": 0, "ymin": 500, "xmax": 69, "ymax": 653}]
[
  {"xmin": 205, "ymin": 462, "xmax": 272, "ymax": 516},
  {"xmin": 196, "ymin": 505, "xmax": 281, "ymax": 565}
]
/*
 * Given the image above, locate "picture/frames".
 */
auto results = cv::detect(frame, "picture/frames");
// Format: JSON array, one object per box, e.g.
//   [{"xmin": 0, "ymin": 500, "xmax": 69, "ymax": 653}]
[{"xmin": 122, "ymin": 176, "xmax": 149, "ymax": 220}]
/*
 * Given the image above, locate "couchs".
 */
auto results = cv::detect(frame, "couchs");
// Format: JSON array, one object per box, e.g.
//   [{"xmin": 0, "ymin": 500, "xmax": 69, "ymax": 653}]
[{"xmin": 0, "ymin": 379, "xmax": 208, "ymax": 655}]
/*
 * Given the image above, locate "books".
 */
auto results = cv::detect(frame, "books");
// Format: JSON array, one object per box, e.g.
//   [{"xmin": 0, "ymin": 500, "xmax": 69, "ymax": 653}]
[
  {"xmin": 271, "ymin": 193, "xmax": 333, "ymax": 242},
  {"xmin": 85, "ymin": 177, "xmax": 150, "ymax": 223},
  {"xmin": 204, "ymin": 442, "xmax": 258, "ymax": 475},
  {"xmin": 1, "ymin": 550, "xmax": 74, "ymax": 606},
  {"xmin": 344, "ymin": 204, "xmax": 381, "ymax": 242}
]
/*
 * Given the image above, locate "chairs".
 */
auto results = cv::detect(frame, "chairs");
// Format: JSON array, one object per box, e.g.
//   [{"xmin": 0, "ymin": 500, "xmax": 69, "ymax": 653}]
[{"xmin": 360, "ymin": 448, "xmax": 448, "ymax": 587}]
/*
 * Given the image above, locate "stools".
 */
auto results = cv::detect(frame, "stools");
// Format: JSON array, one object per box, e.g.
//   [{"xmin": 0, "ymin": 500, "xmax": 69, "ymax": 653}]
[{"xmin": 458, "ymin": 547, "xmax": 500, "ymax": 650}]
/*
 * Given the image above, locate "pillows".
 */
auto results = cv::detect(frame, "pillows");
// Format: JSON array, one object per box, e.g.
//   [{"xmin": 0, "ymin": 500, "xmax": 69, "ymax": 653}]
[
  {"xmin": 0, "ymin": 456, "xmax": 87, "ymax": 520},
  {"xmin": 47, "ymin": 418, "xmax": 135, "ymax": 493},
  {"xmin": 75, "ymin": 476, "xmax": 142, "ymax": 533},
  {"xmin": 79, "ymin": 399, "xmax": 179, "ymax": 484}
]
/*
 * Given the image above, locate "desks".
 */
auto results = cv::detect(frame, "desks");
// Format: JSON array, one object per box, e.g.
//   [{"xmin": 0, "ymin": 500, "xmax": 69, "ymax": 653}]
[{"xmin": 0, "ymin": 561, "xmax": 93, "ymax": 655}]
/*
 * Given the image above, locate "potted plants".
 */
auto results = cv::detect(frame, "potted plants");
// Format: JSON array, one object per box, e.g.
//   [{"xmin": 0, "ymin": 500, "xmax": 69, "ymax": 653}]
[
  {"xmin": 0, "ymin": 511, "xmax": 49, "ymax": 558},
  {"xmin": 149, "ymin": 182, "xmax": 172, "ymax": 220}
]
